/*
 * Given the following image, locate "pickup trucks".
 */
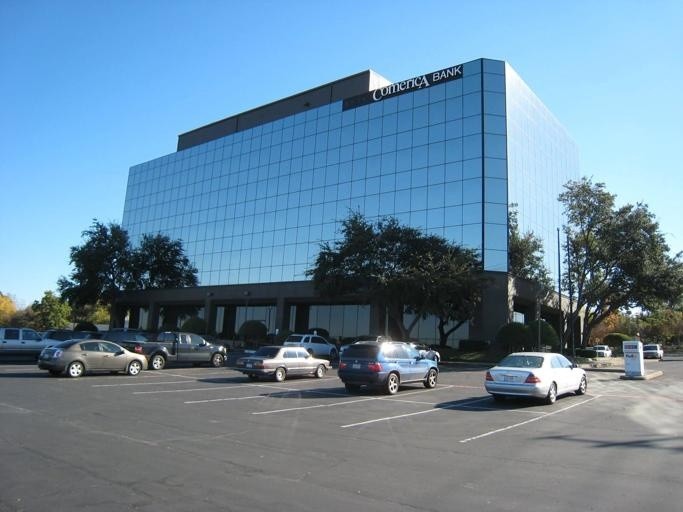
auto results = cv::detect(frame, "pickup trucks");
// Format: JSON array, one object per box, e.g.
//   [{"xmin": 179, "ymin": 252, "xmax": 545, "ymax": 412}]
[{"xmin": 121, "ymin": 331, "xmax": 228, "ymax": 370}]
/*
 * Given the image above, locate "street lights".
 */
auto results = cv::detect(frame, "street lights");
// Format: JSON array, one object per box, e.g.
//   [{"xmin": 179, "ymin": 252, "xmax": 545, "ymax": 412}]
[{"xmin": 534, "ymin": 310, "xmax": 546, "ymax": 351}]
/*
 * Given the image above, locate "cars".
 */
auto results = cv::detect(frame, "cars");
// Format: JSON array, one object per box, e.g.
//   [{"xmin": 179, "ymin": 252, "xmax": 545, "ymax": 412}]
[
  {"xmin": 593, "ymin": 345, "xmax": 612, "ymax": 357},
  {"xmin": 485, "ymin": 352, "xmax": 588, "ymax": 405},
  {"xmin": 234, "ymin": 334, "xmax": 441, "ymax": 395},
  {"xmin": 0, "ymin": 327, "xmax": 149, "ymax": 378},
  {"xmin": 643, "ymin": 344, "xmax": 664, "ymax": 358}
]
